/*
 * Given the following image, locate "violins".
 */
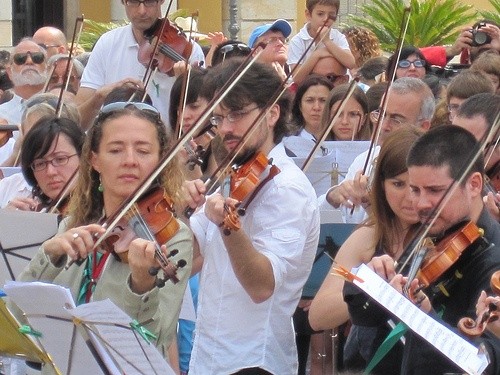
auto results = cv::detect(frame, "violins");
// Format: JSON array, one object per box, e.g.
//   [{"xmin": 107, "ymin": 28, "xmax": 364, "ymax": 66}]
[
  {"xmin": 95, "ymin": 186, "xmax": 180, "ymax": 285},
  {"xmin": 404, "ymin": 220, "xmax": 482, "ymax": 297},
  {"xmin": 458, "ymin": 269, "xmax": 500, "ymax": 336},
  {"xmin": 223, "ymin": 151, "xmax": 269, "ymax": 236},
  {"xmin": 174, "ymin": 128, "xmax": 220, "ymax": 182},
  {"xmin": 137, "ymin": 19, "xmax": 208, "ymax": 77},
  {"xmin": 481, "ymin": 170, "xmax": 500, "ymax": 210}
]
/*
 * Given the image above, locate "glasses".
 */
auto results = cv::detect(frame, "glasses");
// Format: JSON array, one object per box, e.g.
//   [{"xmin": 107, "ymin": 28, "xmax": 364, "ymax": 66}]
[
  {"xmin": 49, "ymin": 76, "xmax": 81, "ymax": 84},
  {"xmin": 369, "ymin": 109, "xmax": 401, "ymax": 129},
  {"xmin": 327, "ymin": 74, "xmax": 345, "ymax": 82},
  {"xmin": 269, "ymin": 37, "xmax": 290, "ymax": 45},
  {"xmin": 13, "ymin": 52, "xmax": 44, "ymax": 65},
  {"xmin": 30, "ymin": 152, "xmax": 81, "ymax": 172},
  {"xmin": 126, "ymin": 0, "xmax": 158, "ymax": 7},
  {"xmin": 39, "ymin": 43, "xmax": 60, "ymax": 49},
  {"xmin": 447, "ymin": 103, "xmax": 460, "ymax": 112},
  {"xmin": 102, "ymin": 101, "xmax": 160, "ymax": 120},
  {"xmin": 218, "ymin": 44, "xmax": 250, "ymax": 54},
  {"xmin": 26, "ymin": 96, "xmax": 59, "ymax": 108},
  {"xmin": 210, "ymin": 106, "xmax": 261, "ymax": 126},
  {"xmin": 398, "ymin": 59, "xmax": 426, "ymax": 68}
]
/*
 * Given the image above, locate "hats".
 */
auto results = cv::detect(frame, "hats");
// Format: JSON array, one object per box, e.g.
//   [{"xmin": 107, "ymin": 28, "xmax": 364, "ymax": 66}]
[
  {"xmin": 248, "ymin": 18, "xmax": 292, "ymax": 48},
  {"xmin": 417, "ymin": 45, "xmax": 446, "ymax": 66}
]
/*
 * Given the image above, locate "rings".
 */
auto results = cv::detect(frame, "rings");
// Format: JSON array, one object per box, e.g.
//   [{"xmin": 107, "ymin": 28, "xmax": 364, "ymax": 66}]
[{"xmin": 72, "ymin": 233, "xmax": 79, "ymax": 243}]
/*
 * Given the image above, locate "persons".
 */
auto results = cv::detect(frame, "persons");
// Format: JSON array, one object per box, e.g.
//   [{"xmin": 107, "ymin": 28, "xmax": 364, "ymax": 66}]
[
  {"xmin": 177, "ymin": 59, "xmax": 321, "ymax": 375},
  {"xmin": 308, "ymin": 125, "xmax": 428, "ymax": 375},
  {"xmin": 12, "ymin": 104, "xmax": 195, "ymax": 375},
  {"xmin": 0, "ymin": 0, "xmax": 500, "ymax": 375}
]
